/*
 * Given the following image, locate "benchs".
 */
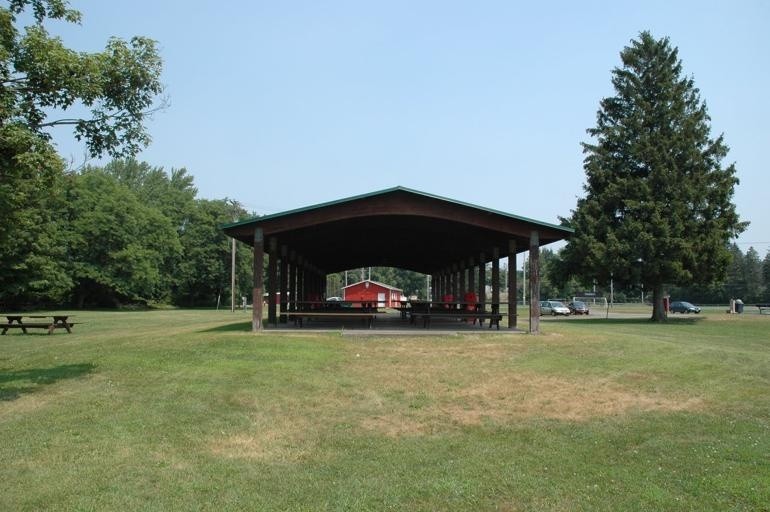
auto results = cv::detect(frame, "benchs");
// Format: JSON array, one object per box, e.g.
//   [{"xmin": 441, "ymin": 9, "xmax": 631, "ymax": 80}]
[
  {"xmin": 1, "ymin": 322, "xmax": 83, "ymax": 334},
  {"xmin": 278, "ymin": 306, "xmax": 519, "ymax": 330}
]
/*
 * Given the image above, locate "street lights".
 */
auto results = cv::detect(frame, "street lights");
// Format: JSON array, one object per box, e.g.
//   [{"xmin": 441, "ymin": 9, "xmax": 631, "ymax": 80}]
[{"xmin": 232, "ymin": 213, "xmax": 240, "ymax": 312}]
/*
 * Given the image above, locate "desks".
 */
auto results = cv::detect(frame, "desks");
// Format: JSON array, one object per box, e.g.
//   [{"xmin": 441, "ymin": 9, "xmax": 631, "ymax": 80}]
[
  {"xmin": 279, "ymin": 300, "xmax": 512, "ymax": 330},
  {"xmin": 0, "ymin": 315, "xmax": 77, "ymax": 334}
]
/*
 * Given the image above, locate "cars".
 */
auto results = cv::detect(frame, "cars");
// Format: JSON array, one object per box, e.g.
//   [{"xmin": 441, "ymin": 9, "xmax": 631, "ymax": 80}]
[
  {"xmin": 669, "ymin": 302, "xmax": 700, "ymax": 314},
  {"xmin": 539, "ymin": 301, "xmax": 589, "ymax": 316},
  {"xmin": 326, "ymin": 297, "xmax": 352, "ymax": 307}
]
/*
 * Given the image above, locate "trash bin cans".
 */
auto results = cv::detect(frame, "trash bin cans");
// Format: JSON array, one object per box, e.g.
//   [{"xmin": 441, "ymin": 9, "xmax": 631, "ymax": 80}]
[
  {"xmin": 443, "ymin": 295, "xmax": 454, "ymax": 309},
  {"xmin": 663, "ymin": 297, "xmax": 669, "ymax": 311},
  {"xmin": 735, "ymin": 299, "xmax": 743, "ymax": 313},
  {"xmin": 464, "ymin": 294, "xmax": 477, "ymax": 320}
]
